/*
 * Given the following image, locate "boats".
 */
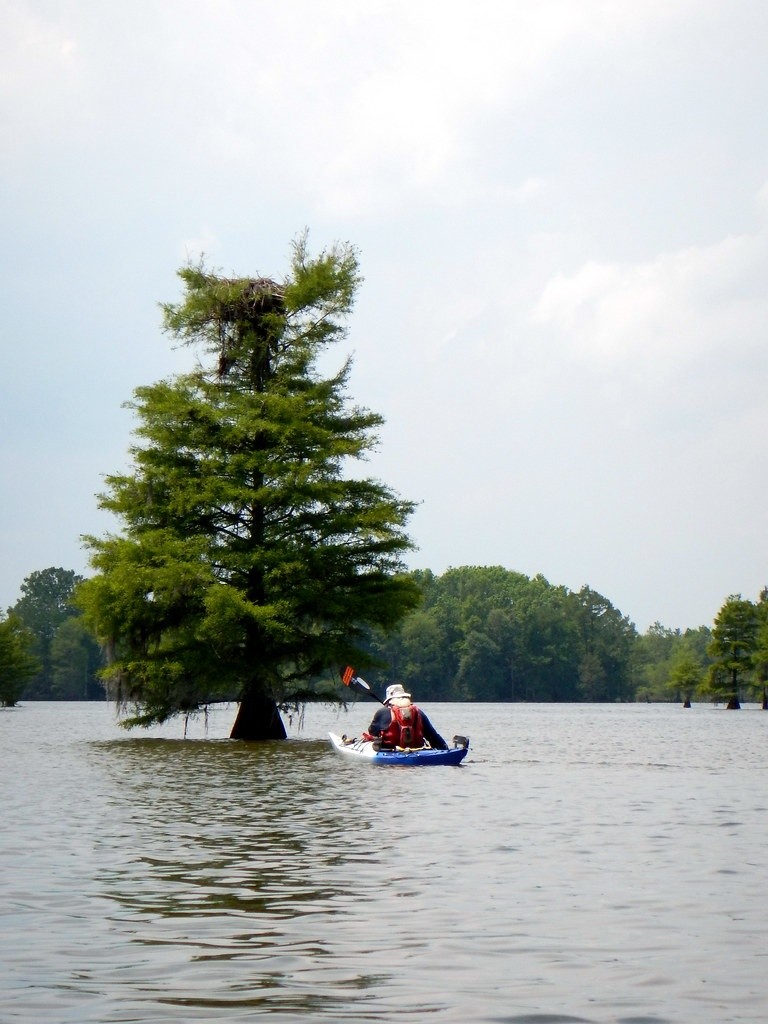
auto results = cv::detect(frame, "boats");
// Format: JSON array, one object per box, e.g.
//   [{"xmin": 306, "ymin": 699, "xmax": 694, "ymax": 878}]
[{"xmin": 325, "ymin": 732, "xmax": 470, "ymax": 766}]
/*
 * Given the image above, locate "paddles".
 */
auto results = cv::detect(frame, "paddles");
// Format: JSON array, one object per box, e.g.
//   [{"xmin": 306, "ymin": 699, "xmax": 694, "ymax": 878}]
[{"xmin": 339, "ymin": 663, "xmax": 430, "ymax": 747}]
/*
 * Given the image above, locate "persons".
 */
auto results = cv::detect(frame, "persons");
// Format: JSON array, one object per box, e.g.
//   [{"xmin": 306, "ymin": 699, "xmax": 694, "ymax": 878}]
[{"xmin": 342, "ymin": 684, "xmax": 449, "ymax": 750}]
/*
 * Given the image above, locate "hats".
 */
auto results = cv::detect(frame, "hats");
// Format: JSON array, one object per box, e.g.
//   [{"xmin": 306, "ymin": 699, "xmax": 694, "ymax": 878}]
[{"xmin": 383, "ymin": 684, "xmax": 411, "ymax": 704}]
